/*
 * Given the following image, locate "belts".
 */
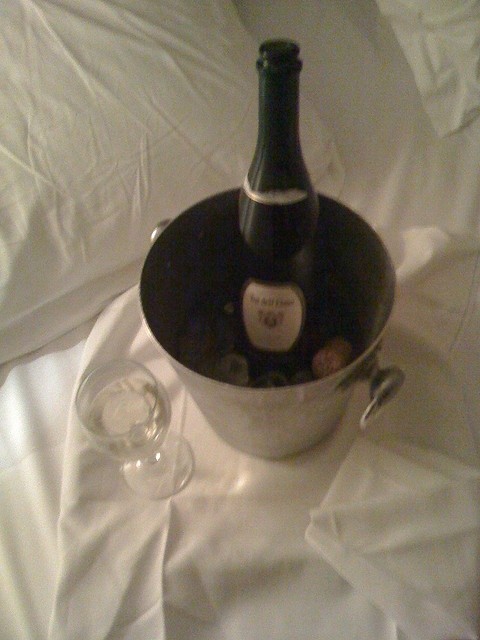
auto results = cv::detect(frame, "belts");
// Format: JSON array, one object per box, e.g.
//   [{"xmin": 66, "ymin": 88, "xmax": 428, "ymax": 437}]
[{"xmin": 74, "ymin": 357, "xmax": 193, "ymax": 499}]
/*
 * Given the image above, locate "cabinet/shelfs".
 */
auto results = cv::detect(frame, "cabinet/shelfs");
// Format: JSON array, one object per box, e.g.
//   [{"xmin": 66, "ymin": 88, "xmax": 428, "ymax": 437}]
[{"xmin": 236, "ymin": 40, "xmax": 320, "ymax": 376}]
